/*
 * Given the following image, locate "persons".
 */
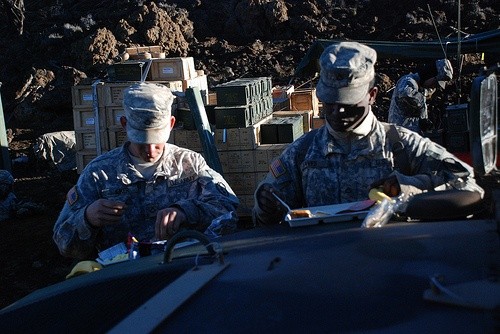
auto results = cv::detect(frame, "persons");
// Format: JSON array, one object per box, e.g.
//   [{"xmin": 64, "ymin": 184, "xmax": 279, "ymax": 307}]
[
  {"xmin": 52, "ymin": 82, "xmax": 241, "ymax": 266},
  {"xmin": 469, "ymin": 52, "xmax": 498, "ymax": 185},
  {"xmin": 0, "ymin": 167, "xmax": 23, "ymax": 285},
  {"xmin": 252, "ymin": 42, "xmax": 475, "ymax": 226},
  {"xmin": 388, "ymin": 57, "xmax": 453, "ymax": 137}
]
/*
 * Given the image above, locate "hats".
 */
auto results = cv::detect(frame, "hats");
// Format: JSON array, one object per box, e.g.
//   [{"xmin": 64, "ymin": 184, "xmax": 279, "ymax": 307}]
[
  {"xmin": 316, "ymin": 42, "xmax": 377, "ymax": 104},
  {"xmin": 123, "ymin": 82, "xmax": 174, "ymax": 144},
  {"xmin": 436, "ymin": 58, "xmax": 453, "ymax": 89}
]
